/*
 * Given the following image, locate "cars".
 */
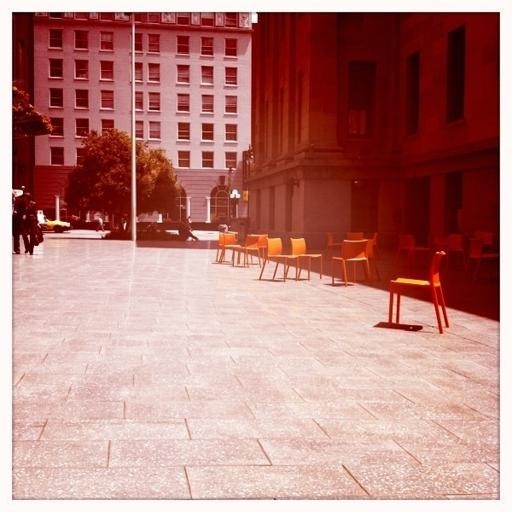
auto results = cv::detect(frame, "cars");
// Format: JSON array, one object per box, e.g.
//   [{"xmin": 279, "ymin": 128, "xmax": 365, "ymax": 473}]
[{"xmin": 40, "ymin": 214, "xmax": 70, "ymax": 233}]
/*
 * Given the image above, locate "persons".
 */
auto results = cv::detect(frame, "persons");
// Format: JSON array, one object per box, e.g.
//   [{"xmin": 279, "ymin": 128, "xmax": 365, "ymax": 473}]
[
  {"xmin": 21, "ymin": 191, "xmax": 41, "ymax": 256},
  {"xmin": 96, "ymin": 214, "xmax": 105, "ymax": 232},
  {"xmin": 12, "ymin": 191, "xmax": 23, "ymax": 255},
  {"xmin": 186, "ymin": 216, "xmax": 191, "ymax": 224},
  {"xmin": 180, "ymin": 218, "xmax": 199, "ymax": 242}
]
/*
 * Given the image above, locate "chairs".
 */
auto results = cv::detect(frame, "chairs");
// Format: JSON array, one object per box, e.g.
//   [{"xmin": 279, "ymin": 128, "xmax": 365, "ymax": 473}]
[{"xmin": 215, "ymin": 232, "xmax": 499, "ymax": 334}]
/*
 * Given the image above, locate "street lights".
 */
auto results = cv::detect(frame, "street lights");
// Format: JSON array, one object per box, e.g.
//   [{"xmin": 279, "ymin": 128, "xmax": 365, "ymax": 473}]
[{"xmin": 230, "ymin": 189, "xmax": 241, "ymax": 218}]
